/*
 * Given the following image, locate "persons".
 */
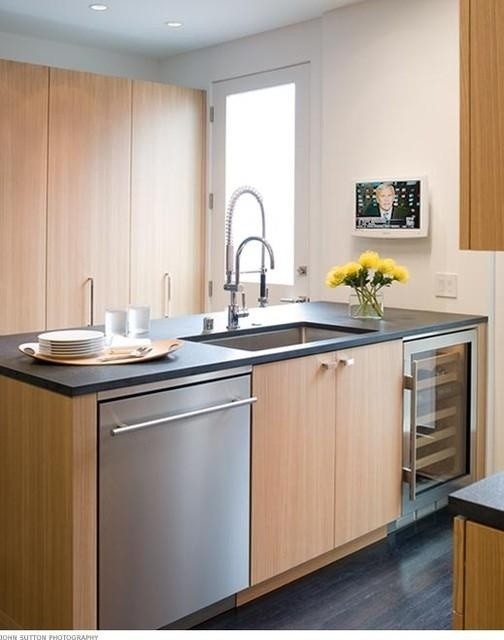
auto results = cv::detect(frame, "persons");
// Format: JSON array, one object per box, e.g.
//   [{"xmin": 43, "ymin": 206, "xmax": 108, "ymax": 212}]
[{"xmin": 359, "ymin": 184, "xmax": 410, "ymax": 226}]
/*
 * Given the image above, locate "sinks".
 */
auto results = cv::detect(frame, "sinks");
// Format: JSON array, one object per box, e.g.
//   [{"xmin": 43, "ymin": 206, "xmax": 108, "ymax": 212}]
[{"xmin": 178, "ymin": 321, "xmax": 379, "ymax": 351}]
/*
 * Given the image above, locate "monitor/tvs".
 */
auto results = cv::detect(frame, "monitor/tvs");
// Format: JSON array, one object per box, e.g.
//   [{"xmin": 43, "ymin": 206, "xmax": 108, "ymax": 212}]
[{"xmin": 352, "ymin": 177, "xmax": 429, "ymax": 239}]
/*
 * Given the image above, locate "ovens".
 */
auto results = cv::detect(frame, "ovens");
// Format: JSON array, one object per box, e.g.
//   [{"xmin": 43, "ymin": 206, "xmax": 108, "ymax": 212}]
[{"xmin": 96, "ymin": 366, "xmax": 258, "ymax": 630}]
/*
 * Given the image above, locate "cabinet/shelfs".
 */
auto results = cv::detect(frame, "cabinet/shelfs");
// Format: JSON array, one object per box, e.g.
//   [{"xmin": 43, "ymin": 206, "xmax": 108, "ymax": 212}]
[
  {"xmin": 457, "ymin": 0, "xmax": 503, "ymax": 252},
  {"xmin": 129, "ymin": 79, "xmax": 208, "ymax": 321},
  {"xmin": 0, "ymin": 58, "xmax": 46, "ymax": 336},
  {"xmin": 46, "ymin": 67, "xmax": 134, "ymax": 333},
  {"xmin": 445, "ymin": 471, "xmax": 504, "ymax": 628}
]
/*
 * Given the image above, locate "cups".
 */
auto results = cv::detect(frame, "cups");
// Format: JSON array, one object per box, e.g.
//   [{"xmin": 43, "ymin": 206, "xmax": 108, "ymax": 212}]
[
  {"xmin": 105, "ymin": 310, "xmax": 128, "ymax": 337},
  {"xmin": 127, "ymin": 304, "xmax": 149, "ymax": 334}
]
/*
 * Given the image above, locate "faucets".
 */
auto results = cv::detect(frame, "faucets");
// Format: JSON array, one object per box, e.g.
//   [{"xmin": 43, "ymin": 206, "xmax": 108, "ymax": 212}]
[{"xmin": 235, "ymin": 236, "xmax": 275, "ymax": 283}]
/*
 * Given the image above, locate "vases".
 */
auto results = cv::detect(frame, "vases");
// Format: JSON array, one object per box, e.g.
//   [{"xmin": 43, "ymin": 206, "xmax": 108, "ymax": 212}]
[{"xmin": 349, "ymin": 293, "xmax": 385, "ymax": 319}]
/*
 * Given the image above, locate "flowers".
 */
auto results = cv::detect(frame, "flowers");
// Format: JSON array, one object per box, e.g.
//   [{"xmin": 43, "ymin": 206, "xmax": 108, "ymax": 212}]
[{"xmin": 326, "ymin": 248, "xmax": 409, "ymax": 318}]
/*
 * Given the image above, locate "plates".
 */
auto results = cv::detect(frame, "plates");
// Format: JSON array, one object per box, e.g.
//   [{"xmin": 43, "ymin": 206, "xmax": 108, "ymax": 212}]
[{"xmin": 36, "ymin": 329, "xmax": 106, "ymax": 358}]
